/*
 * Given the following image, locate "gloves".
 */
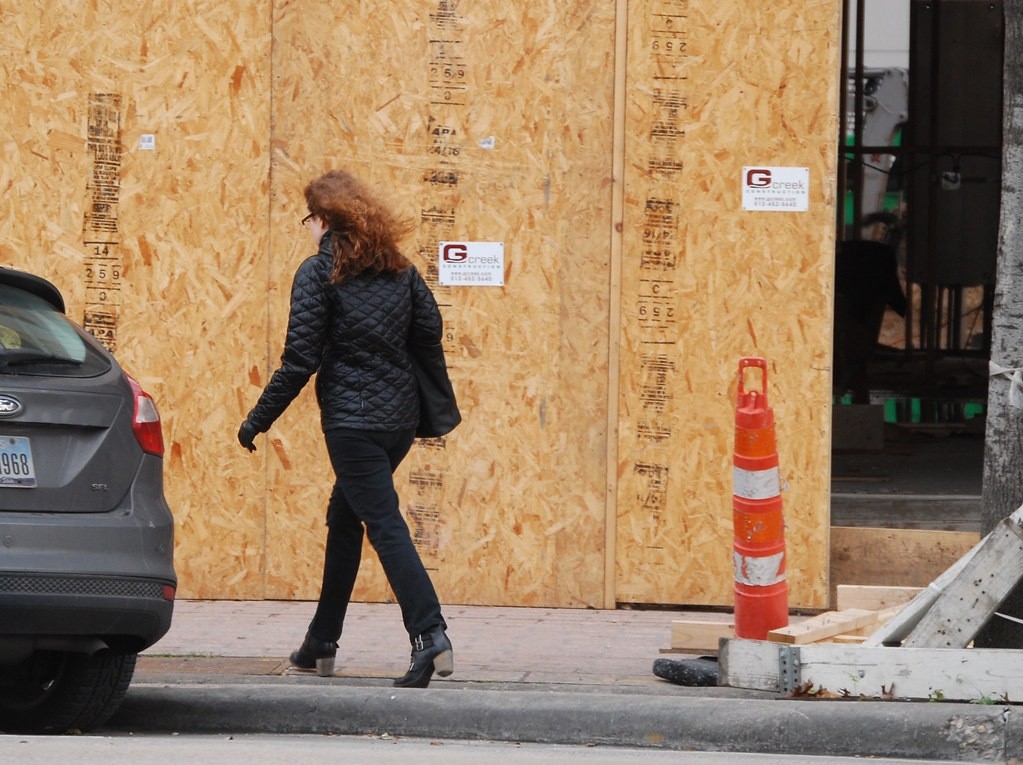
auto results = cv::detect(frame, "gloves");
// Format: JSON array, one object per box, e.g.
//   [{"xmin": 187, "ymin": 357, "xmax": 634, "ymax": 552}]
[{"xmin": 238, "ymin": 421, "xmax": 260, "ymax": 453}]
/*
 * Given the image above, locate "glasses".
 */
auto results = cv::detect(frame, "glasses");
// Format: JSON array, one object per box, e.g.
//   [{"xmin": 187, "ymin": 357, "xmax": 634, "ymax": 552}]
[{"xmin": 302, "ymin": 212, "xmax": 314, "ymax": 230}]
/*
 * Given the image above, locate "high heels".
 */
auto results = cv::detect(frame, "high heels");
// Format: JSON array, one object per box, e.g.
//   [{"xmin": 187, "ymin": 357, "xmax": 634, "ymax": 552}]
[
  {"xmin": 393, "ymin": 624, "xmax": 454, "ymax": 688},
  {"xmin": 289, "ymin": 630, "xmax": 336, "ymax": 676}
]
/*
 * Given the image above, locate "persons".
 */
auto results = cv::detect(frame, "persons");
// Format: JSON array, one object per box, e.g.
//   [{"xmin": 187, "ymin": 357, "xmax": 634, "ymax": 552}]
[{"xmin": 237, "ymin": 170, "xmax": 467, "ymax": 689}]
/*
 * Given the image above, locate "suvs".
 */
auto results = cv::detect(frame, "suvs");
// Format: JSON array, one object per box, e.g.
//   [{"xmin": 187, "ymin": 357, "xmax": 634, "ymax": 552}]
[{"xmin": 1, "ymin": 265, "xmax": 179, "ymax": 734}]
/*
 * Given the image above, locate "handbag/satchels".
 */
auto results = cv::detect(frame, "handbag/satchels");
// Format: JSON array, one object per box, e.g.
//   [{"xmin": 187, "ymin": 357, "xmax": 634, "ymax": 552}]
[{"xmin": 412, "ymin": 340, "xmax": 462, "ymax": 437}]
